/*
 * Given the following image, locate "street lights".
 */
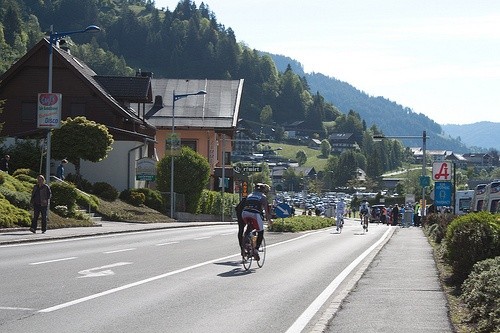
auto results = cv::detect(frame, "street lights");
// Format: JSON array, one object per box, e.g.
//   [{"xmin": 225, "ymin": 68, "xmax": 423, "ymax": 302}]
[
  {"xmin": 372, "ymin": 129, "xmax": 430, "ymax": 217},
  {"xmin": 45, "ymin": 25, "xmax": 101, "ymax": 219},
  {"xmin": 219, "ymin": 134, "xmax": 269, "ymax": 222},
  {"xmin": 250, "ymin": 147, "xmax": 283, "ymax": 193},
  {"xmin": 170, "ymin": 89, "xmax": 208, "ymax": 218}
]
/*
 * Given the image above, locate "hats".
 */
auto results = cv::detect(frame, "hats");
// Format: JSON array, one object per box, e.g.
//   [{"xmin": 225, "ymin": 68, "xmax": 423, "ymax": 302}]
[{"xmin": 254, "ymin": 182, "xmax": 264, "ymax": 190}]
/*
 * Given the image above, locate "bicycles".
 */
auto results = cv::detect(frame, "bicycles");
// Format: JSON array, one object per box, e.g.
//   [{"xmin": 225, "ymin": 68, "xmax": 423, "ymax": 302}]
[
  {"xmin": 240, "ymin": 218, "xmax": 269, "ymax": 270},
  {"xmin": 338, "ymin": 217, "xmax": 344, "ymax": 234},
  {"xmin": 359, "ymin": 213, "xmax": 368, "ymax": 232}
]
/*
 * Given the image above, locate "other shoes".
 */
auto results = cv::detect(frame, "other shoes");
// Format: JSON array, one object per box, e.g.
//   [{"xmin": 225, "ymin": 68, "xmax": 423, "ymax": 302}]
[{"xmin": 253, "ymin": 248, "xmax": 260, "ymax": 261}]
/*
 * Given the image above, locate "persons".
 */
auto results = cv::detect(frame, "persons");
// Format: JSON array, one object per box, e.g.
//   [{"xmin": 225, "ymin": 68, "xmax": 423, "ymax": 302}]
[
  {"xmin": 235, "ymin": 193, "xmax": 254, "ymax": 256},
  {"xmin": 29, "ymin": 174, "xmax": 51, "ymax": 233},
  {"xmin": 242, "ymin": 183, "xmax": 272, "ymax": 260},
  {"xmin": 57, "ymin": 159, "xmax": 68, "ymax": 180},
  {"xmin": 290, "ymin": 197, "xmax": 423, "ymax": 231},
  {"xmin": 0, "ymin": 154, "xmax": 10, "ymax": 173}
]
